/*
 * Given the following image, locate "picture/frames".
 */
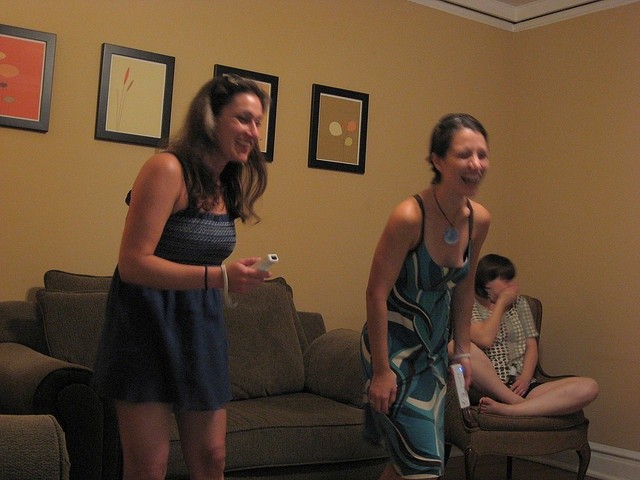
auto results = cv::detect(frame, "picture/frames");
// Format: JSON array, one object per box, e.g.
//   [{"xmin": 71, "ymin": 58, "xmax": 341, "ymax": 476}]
[
  {"xmin": 306, "ymin": 83, "xmax": 370, "ymax": 174},
  {"xmin": 0, "ymin": 22, "xmax": 58, "ymax": 133},
  {"xmin": 213, "ymin": 63, "xmax": 280, "ymax": 162},
  {"xmin": 94, "ymin": 42, "xmax": 176, "ymax": 148}
]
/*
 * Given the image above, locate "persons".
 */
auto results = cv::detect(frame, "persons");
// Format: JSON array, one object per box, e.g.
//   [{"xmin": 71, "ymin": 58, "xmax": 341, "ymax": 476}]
[
  {"xmin": 89, "ymin": 72, "xmax": 273, "ymax": 480},
  {"xmin": 359, "ymin": 112, "xmax": 492, "ymax": 479},
  {"xmin": 448, "ymin": 253, "xmax": 599, "ymax": 417}
]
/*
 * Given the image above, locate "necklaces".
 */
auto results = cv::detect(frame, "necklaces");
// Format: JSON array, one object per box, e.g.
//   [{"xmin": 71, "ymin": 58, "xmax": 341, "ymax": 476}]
[{"xmin": 433, "ymin": 187, "xmax": 460, "ymax": 245}]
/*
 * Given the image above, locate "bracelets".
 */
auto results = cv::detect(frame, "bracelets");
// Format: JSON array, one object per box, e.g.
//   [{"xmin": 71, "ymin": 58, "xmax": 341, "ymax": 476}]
[
  {"xmin": 452, "ymin": 353, "xmax": 471, "ymax": 359},
  {"xmin": 204, "ymin": 265, "xmax": 208, "ymax": 290}
]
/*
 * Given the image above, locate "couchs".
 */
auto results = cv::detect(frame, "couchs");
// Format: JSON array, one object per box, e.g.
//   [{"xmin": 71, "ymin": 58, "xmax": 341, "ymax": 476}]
[
  {"xmin": 1, "ymin": 413, "xmax": 72, "ymax": 480},
  {"xmin": 1, "ymin": 269, "xmax": 394, "ymax": 478},
  {"xmin": 444, "ymin": 290, "xmax": 592, "ymax": 480}
]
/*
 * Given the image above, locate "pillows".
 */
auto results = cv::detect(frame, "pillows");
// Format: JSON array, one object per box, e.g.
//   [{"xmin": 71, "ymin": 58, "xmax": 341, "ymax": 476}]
[
  {"xmin": 35, "ymin": 288, "xmax": 111, "ymax": 368},
  {"xmin": 218, "ymin": 279, "xmax": 308, "ymax": 403}
]
